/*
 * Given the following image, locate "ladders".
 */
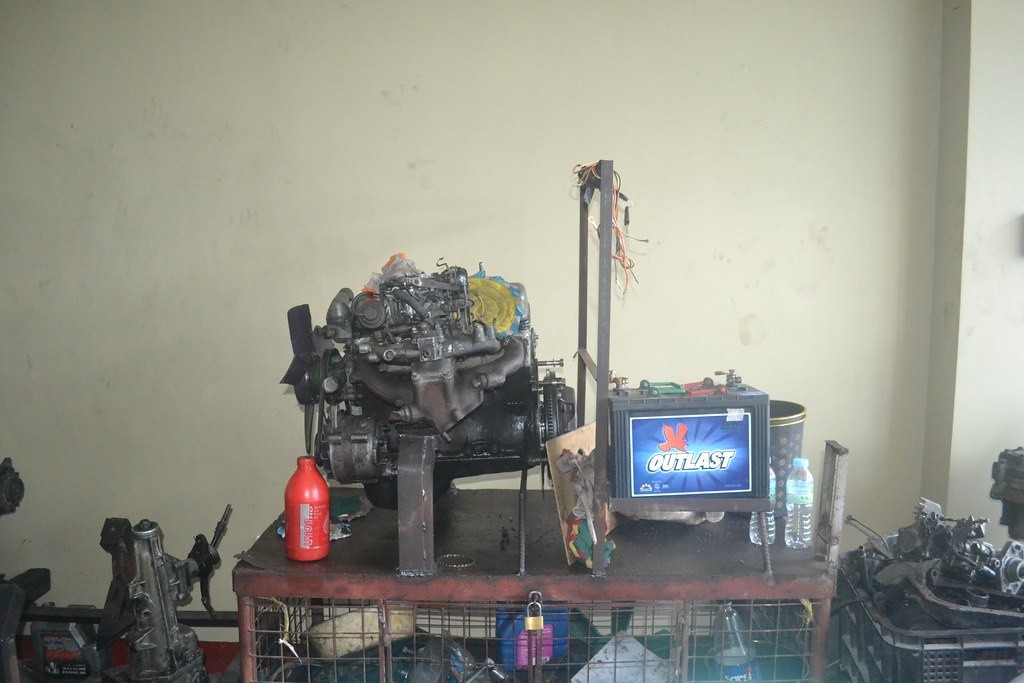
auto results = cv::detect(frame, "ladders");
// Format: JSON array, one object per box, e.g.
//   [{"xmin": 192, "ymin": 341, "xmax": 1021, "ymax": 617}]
[{"xmin": 805, "ymin": 435, "xmax": 850, "ymax": 683}]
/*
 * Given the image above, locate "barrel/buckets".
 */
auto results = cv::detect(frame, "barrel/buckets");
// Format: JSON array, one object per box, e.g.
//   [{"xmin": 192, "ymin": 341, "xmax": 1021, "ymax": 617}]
[
  {"xmin": 770, "ymin": 400, "xmax": 808, "ymax": 424},
  {"xmin": 744, "ymin": 401, "xmax": 806, "ymax": 518}
]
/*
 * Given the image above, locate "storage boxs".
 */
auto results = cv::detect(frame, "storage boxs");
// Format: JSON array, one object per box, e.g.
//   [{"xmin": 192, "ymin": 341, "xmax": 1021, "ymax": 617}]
[{"xmin": 836, "ymin": 560, "xmax": 1024, "ymax": 683}]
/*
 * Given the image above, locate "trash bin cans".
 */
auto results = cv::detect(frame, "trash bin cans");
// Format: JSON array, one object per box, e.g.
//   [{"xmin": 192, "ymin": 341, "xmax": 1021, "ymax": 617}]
[{"xmin": 769, "ymin": 401, "xmax": 809, "ymax": 520}]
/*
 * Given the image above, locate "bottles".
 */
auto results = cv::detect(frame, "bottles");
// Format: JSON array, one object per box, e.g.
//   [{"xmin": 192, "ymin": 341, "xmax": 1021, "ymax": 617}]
[
  {"xmin": 784, "ymin": 457, "xmax": 814, "ymax": 549},
  {"xmin": 284, "ymin": 456, "xmax": 330, "ymax": 561},
  {"xmin": 714, "ymin": 600, "xmax": 760, "ymax": 683},
  {"xmin": 749, "ymin": 457, "xmax": 776, "ymax": 545}
]
